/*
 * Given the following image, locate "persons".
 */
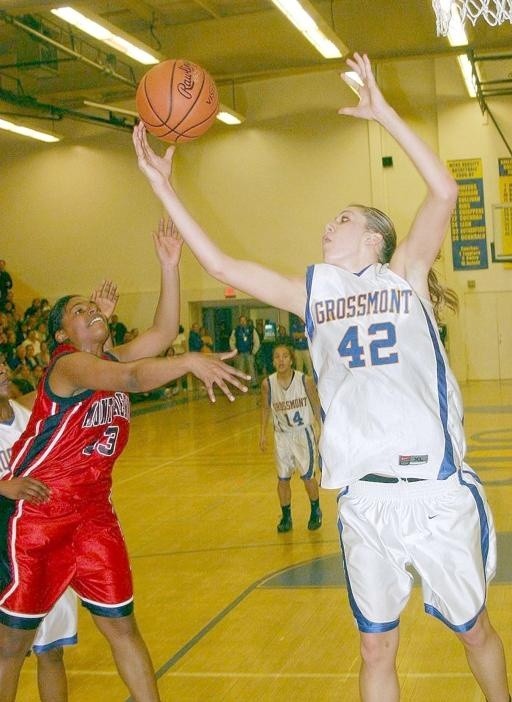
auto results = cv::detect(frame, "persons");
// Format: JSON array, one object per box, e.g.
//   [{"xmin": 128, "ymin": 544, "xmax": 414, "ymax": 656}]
[
  {"xmin": 259, "ymin": 343, "xmax": 326, "ymax": 533},
  {"xmin": 130, "ymin": 49, "xmax": 512, "ymax": 701},
  {"xmin": 2, "ymin": 215, "xmax": 252, "ymax": 700},
  {"xmin": 436, "ymin": 319, "xmax": 445, "ymax": 347},
  {"xmin": 0, "ymin": 277, "xmax": 118, "ymax": 701}
]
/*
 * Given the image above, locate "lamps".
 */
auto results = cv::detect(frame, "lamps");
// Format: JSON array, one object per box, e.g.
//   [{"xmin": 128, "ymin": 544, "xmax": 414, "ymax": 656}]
[
  {"xmin": 0, "ymin": 0, "xmax": 246, "ymax": 140},
  {"xmin": 270, "ymin": 0, "xmax": 351, "ymax": 61}
]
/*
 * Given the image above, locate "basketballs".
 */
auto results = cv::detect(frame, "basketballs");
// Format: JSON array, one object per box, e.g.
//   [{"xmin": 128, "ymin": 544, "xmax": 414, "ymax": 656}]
[{"xmin": 135, "ymin": 59, "xmax": 220, "ymax": 144}]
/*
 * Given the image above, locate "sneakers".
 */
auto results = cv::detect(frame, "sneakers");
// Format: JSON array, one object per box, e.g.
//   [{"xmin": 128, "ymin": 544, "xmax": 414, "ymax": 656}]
[
  {"xmin": 308, "ymin": 512, "xmax": 322, "ymax": 530},
  {"xmin": 277, "ymin": 518, "xmax": 292, "ymax": 531}
]
